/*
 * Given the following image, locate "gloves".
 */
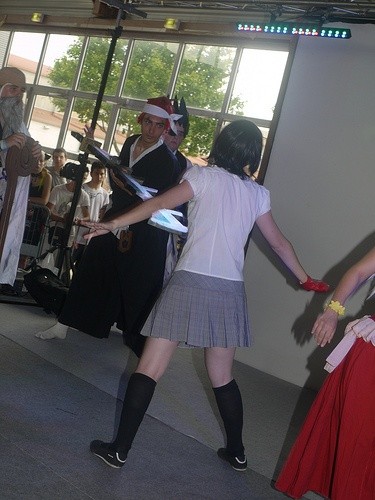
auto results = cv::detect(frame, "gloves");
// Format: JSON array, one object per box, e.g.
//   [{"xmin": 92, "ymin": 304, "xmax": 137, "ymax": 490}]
[{"xmin": 300, "ymin": 276, "xmax": 332, "ymax": 293}]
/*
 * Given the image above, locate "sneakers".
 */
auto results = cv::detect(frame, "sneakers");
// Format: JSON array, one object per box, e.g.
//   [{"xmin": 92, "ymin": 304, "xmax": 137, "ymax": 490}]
[
  {"xmin": 219, "ymin": 448, "xmax": 248, "ymax": 471},
  {"xmin": 90, "ymin": 441, "xmax": 126, "ymax": 468}
]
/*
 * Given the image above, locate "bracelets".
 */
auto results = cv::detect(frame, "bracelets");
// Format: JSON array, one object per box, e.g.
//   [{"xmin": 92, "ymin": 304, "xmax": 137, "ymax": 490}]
[{"xmin": 325, "ymin": 302, "xmax": 344, "ymax": 315}]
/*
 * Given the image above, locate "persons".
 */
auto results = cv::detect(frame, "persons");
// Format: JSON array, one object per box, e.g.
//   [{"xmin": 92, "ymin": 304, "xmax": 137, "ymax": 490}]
[
  {"xmin": 0, "ymin": 68, "xmax": 41, "ymax": 284},
  {"xmin": 80, "ymin": 122, "xmax": 329, "ymax": 470},
  {"xmin": 276, "ymin": 247, "xmax": 375, "ymax": 500},
  {"xmin": 0, "ymin": 96, "xmax": 193, "ymax": 359}
]
